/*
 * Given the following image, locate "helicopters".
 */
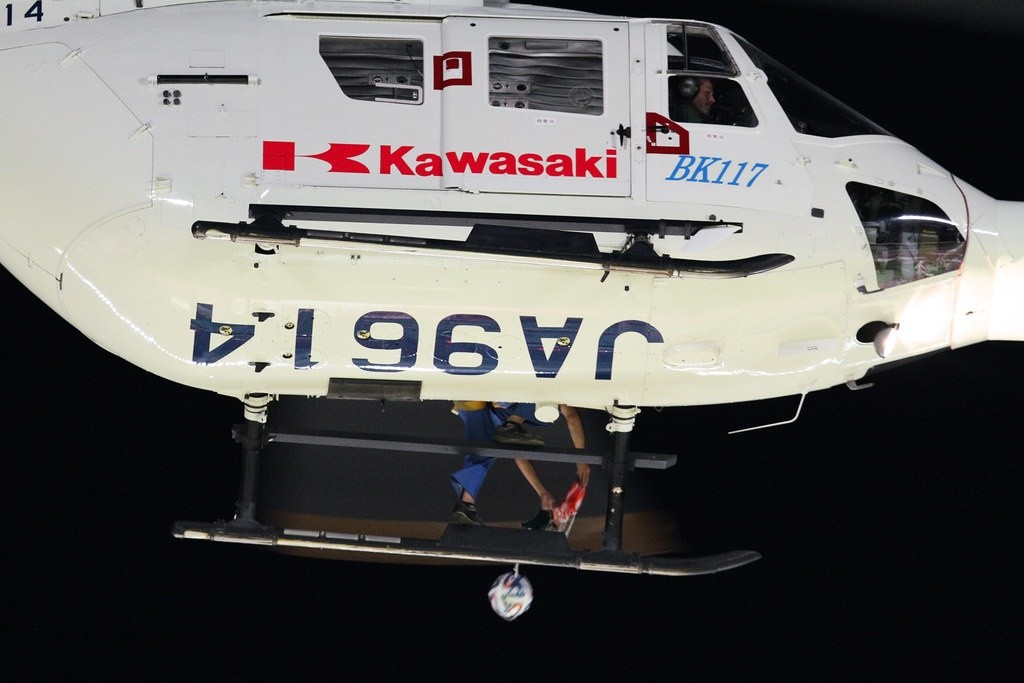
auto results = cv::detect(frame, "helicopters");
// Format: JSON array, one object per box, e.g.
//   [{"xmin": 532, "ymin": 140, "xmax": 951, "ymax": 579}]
[{"xmin": 2, "ymin": 0, "xmax": 1024, "ymax": 578}]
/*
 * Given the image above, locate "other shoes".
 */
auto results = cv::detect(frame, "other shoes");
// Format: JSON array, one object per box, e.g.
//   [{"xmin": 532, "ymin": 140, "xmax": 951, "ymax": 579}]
[
  {"xmin": 493, "ymin": 420, "xmax": 544, "ymax": 445},
  {"xmin": 453, "ymin": 501, "xmax": 485, "ymax": 526}
]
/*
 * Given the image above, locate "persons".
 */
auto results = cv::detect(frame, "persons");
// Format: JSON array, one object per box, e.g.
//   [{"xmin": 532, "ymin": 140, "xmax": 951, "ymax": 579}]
[
  {"xmin": 669, "ymin": 77, "xmax": 714, "ymax": 123},
  {"xmin": 448, "ymin": 400, "xmax": 590, "ymax": 532}
]
